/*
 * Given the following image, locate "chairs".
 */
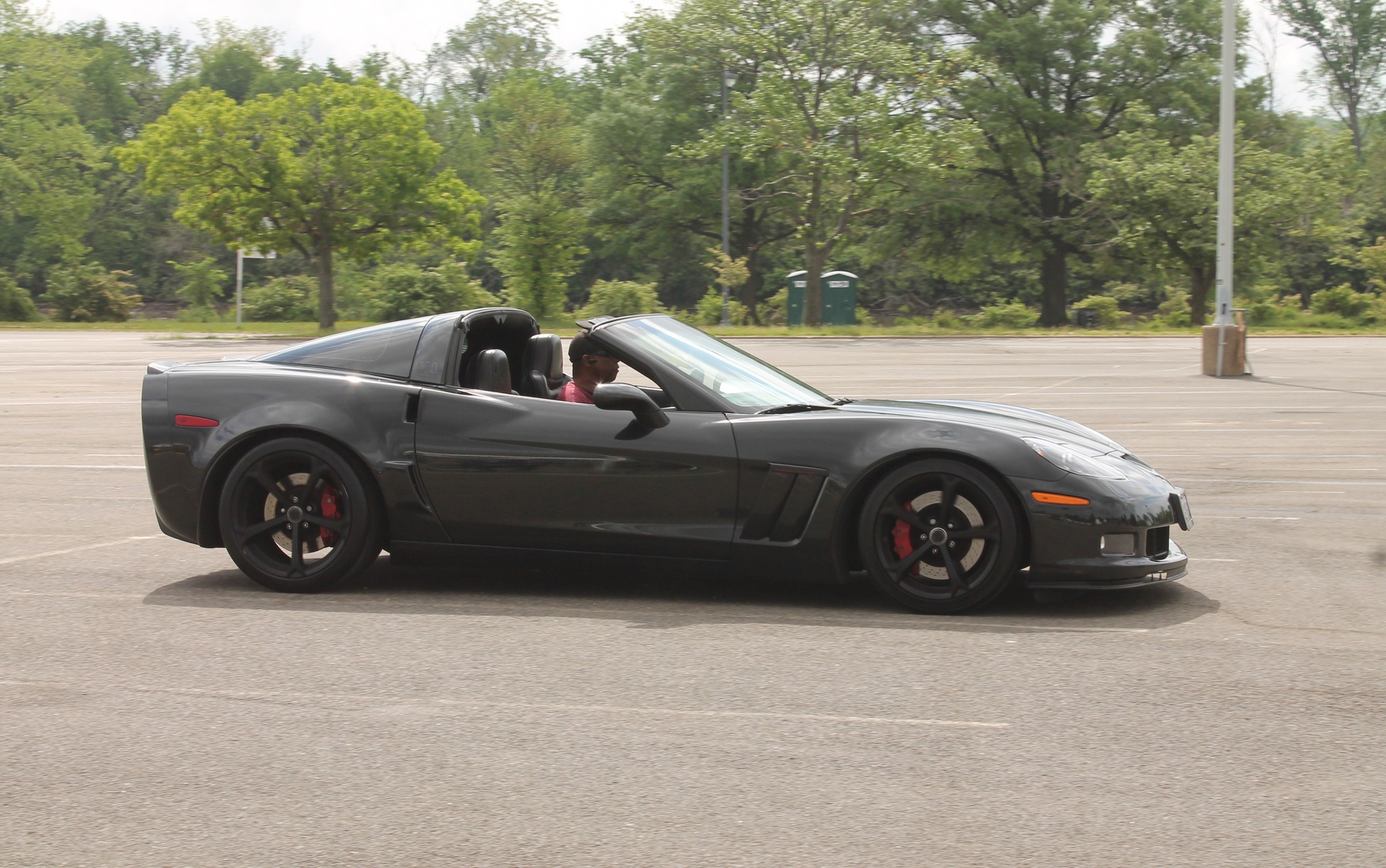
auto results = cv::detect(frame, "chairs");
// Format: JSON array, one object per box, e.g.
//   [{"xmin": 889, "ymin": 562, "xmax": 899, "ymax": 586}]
[
  {"xmin": 520, "ymin": 335, "xmax": 575, "ymax": 400},
  {"xmin": 464, "ymin": 348, "xmax": 518, "ymax": 396}
]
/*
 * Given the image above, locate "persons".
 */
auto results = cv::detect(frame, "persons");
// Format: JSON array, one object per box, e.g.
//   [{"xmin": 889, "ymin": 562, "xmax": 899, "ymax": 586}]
[{"xmin": 555, "ymin": 330, "xmax": 620, "ymax": 405}]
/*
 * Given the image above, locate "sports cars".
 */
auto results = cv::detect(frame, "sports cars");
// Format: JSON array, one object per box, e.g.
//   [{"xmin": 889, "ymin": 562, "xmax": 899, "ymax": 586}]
[{"xmin": 141, "ymin": 304, "xmax": 1195, "ymax": 612}]
